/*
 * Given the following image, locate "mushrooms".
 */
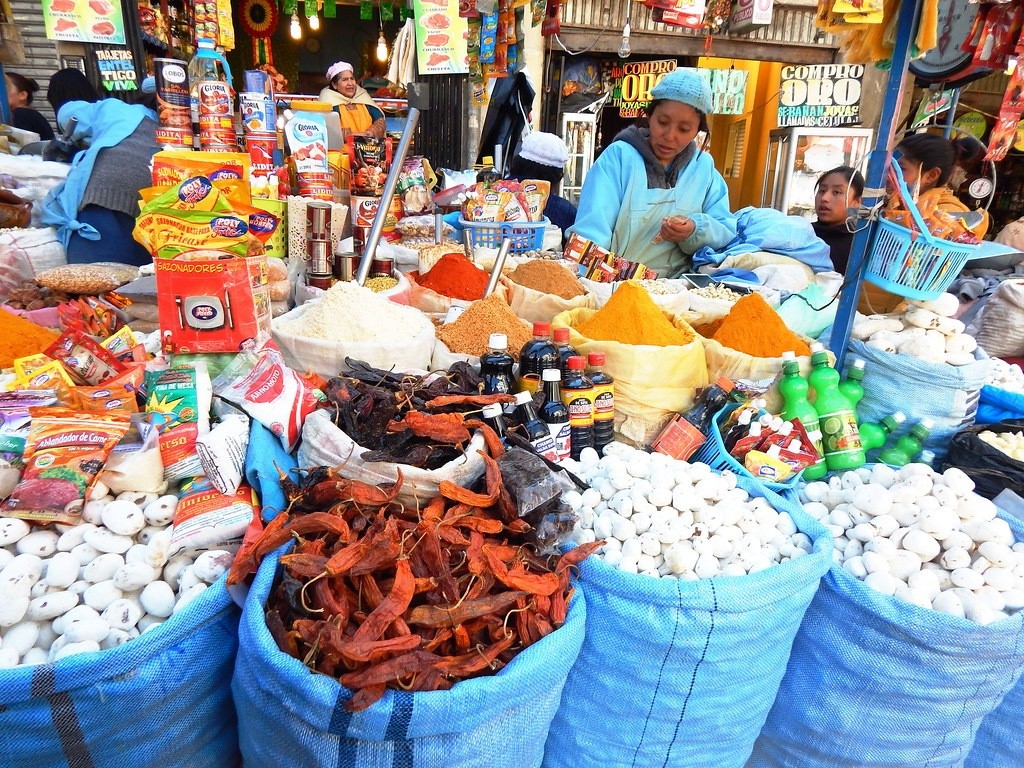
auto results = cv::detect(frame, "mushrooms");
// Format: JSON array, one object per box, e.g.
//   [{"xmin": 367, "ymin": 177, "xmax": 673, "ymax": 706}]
[
  {"xmin": 0, "ymin": 478, "xmax": 236, "ymax": 674},
  {"xmin": 844, "ymin": 292, "xmax": 1024, "ymax": 398},
  {"xmin": 555, "ymin": 443, "xmax": 1024, "ymax": 627}
]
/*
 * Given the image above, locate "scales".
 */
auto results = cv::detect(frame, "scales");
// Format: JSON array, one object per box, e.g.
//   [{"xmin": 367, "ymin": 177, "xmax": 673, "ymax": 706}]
[
  {"xmin": 907, "ymin": 0, "xmax": 997, "ymax": 127},
  {"xmin": 968, "ymin": 175, "xmax": 993, "ymax": 210}
]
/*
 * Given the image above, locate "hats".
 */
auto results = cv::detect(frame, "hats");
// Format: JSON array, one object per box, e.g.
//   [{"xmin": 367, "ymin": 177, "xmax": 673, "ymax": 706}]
[
  {"xmin": 650, "ymin": 67, "xmax": 714, "ymax": 115},
  {"xmin": 325, "ymin": 61, "xmax": 353, "ymax": 82},
  {"xmin": 519, "ymin": 131, "xmax": 569, "ymax": 168}
]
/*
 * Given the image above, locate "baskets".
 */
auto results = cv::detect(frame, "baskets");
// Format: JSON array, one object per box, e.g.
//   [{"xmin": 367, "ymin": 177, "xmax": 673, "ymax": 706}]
[{"xmin": 864, "ymin": 154, "xmax": 979, "ymax": 303}]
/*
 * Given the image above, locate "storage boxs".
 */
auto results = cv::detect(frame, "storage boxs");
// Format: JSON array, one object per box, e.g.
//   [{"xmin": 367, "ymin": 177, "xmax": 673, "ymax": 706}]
[
  {"xmin": 253, "ymin": 197, "xmax": 287, "ymax": 258},
  {"xmin": 154, "ymin": 257, "xmax": 270, "ymax": 355}
]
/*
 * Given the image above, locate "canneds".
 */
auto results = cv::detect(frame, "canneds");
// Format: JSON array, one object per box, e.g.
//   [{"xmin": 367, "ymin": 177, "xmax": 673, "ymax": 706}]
[
  {"xmin": 152, "ymin": 57, "xmax": 334, "ymax": 201},
  {"xmin": 306, "ymin": 201, "xmax": 395, "ymax": 290}
]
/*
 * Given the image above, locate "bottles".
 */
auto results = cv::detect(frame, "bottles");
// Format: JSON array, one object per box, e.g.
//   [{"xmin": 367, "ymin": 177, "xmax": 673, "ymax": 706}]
[
  {"xmin": 187, "ymin": 38, "xmax": 234, "ymax": 123},
  {"xmin": 478, "ymin": 321, "xmax": 615, "ymax": 472},
  {"xmin": 476, "ymin": 156, "xmax": 503, "ymax": 183},
  {"xmin": 643, "ymin": 342, "xmax": 935, "ymax": 481}
]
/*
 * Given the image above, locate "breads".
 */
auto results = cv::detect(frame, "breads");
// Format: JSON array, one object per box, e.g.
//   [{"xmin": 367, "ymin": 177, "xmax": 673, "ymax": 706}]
[{"xmin": 267, "ymin": 257, "xmax": 290, "ymax": 317}]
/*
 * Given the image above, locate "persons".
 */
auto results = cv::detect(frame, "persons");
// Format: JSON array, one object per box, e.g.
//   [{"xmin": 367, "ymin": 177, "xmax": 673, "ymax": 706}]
[
  {"xmin": 3, "ymin": 68, "xmax": 159, "ymax": 267},
  {"xmin": 564, "ymin": 67, "xmax": 738, "ymax": 282},
  {"xmin": 320, "ymin": 60, "xmax": 386, "ymax": 138},
  {"xmin": 812, "ymin": 132, "xmax": 1024, "ymax": 314},
  {"xmin": 505, "ymin": 131, "xmax": 577, "ymax": 247}
]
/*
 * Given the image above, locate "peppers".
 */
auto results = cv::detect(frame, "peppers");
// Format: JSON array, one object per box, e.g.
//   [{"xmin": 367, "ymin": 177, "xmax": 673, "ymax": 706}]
[{"xmin": 226, "ymin": 354, "xmax": 610, "ymax": 715}]
